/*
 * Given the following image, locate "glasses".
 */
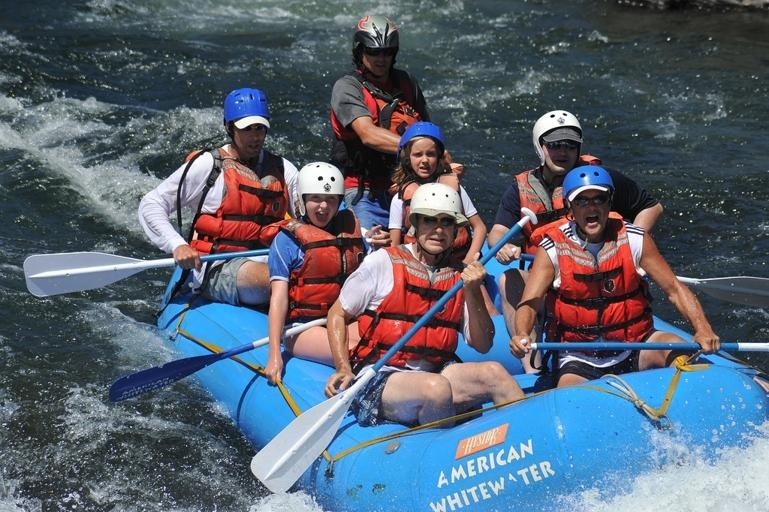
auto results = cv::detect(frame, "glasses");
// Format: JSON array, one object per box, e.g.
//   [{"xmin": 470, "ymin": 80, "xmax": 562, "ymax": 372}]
[
  {"xmin": 543, "ymin": 141, "xmax": 578, "ymax": 152},
  {"xmin": 574, "ymin": 197, "xmax": 606, "ymax": 206},
  {"xmin": 243, "ymin": 125, "xmax": 265, "ymax": 131},
  {"xmin": 546, "ymin": 141, "xmax": 578, "ymax": 149},
  {"xmin": 365, "ymin": 48, "xmax": 393, "ymax": 56},
  {"xmin": 424, "ymin": 216, "xmax": 453, "ymax": 228},
  {"xmin": 422, "ymin": 218, "xmax": 454, "ymax": 226}
]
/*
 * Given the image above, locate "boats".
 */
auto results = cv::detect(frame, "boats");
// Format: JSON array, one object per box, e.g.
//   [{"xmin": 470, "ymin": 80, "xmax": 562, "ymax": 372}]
[{"xmin": 156, "ymin": 237, "xmax": 769, "ymax": 512}]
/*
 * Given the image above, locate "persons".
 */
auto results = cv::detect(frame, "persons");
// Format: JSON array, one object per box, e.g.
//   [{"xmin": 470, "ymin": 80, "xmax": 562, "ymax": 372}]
[
  {"xmin": 325, "ymin": 14, "xmax": 450, "ymax": 260},
  {"xmin": 136, "ymin": 85, "xmax": 392, "ymax": 308},
  {"xmin": 262, "ymin": 160, "xmax": 371, "ymax": 387},
  {"xmin": 386, "ymin": 121, "xmax": 487, "ymax": 272},
  {"xmin": 320, "ymin": 182, "xmax": 526, "ymax": 429},
  {"xmin": 477, "ymin": 110, "xmax": 665, "ymax": 374},
  {"xmin": 500, "ymin": 164, "xmax": 720, "ymax": 387}
]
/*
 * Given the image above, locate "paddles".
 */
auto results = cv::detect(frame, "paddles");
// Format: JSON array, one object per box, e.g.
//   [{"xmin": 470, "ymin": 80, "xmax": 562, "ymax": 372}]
[
  {"xmin": 109, "ymin": 317, "xmax": 325, "ymax": 400},
  {"xmin": 509, "ymin": 247, "xmax": 768, "ymax": 316},
  {"xmin": 250, "ymin": 208, "xmax": 538, "ymax": 493},
  {"xmin": 22, "ymin": 232, "xmax": 379, "ymax": 297}
]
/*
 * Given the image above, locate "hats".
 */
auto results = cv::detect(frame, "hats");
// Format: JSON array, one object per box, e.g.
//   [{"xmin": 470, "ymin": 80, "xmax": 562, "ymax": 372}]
[
  {"xmin": 542, "ymin": 128, "xmax": 582, "ymax": 143},
  {"xmin": 408, "ymin": 210, "xmax": 469, "ymax": 229},
  {"xmin": 567, "ymin": 185, "xmax": 609, "ymax": 202},
  {"xmin": 234, "ymin": 116, "xmax": 270, "ymax": 130}
]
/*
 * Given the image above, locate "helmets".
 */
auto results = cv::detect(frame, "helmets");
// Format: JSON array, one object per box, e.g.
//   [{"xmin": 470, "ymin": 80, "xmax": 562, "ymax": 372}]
[
  {"xmin": 224, "ymin": 88, "xmax": 270, "ymax": 122},
  {"xmin": 409, "ymin": 183, "xmax": 462, "ymax": 215},
  {"xmin": 353, "ymin": 15, "xmax": 398, "ymax": 52},
  {"xmin": 532, "ymin": 110, "xmax": 582, "ymax": 166},
  {"xmin": 296, "ymin": 162, "xmax": 344, "ymax": 217},
  {"xmin": 399, "ymin": 122, "xmax": 445, "ymax": 150},
  {"xmin": 562, "ymin": 165, "xmax": 615, "ymax": 199}
]
